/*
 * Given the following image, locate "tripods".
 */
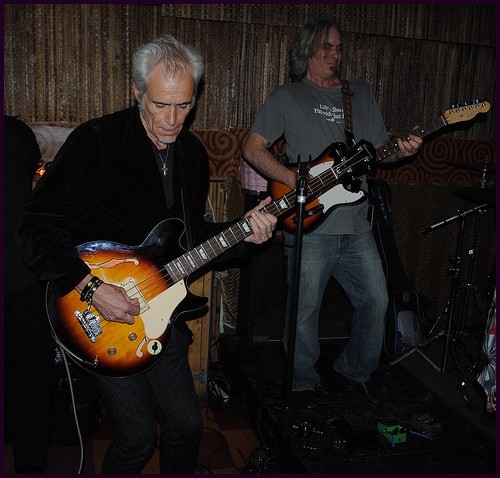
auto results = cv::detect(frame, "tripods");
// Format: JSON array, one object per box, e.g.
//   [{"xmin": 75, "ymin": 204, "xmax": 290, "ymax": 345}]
[{"xmin": 388, "ymin": 170, "xmax": 487, "ymax": 409}]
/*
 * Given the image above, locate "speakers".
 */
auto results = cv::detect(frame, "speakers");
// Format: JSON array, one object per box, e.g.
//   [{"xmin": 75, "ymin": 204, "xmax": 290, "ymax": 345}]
[{"xmin": 238, "ymin": 193, "xmax": 356, "ymax": 350}]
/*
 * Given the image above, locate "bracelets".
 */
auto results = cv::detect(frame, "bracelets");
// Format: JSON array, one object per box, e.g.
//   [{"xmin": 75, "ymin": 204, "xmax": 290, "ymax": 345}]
[
  {"xmin": 85, "ymin": 280, "xmax": 104, "ymax": 302},
  {"xmin": 80, "ymin": 276, "xmax": 99, "ymax": 302}
]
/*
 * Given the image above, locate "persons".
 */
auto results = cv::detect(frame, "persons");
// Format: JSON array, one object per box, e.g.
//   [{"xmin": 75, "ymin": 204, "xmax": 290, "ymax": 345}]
[
  {"xmin": 4, "ymin": 116, "xmax": 57, "ymax": 472},
  {"xmin": 243, "ymin": 17, "xmax": 422, "ymax": 414},
  {"xmin": 16, "ymin": 34, "xmax": 277, "ymax": 474}
]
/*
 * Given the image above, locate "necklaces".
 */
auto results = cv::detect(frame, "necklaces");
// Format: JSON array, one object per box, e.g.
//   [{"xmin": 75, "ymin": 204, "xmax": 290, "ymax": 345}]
[{"xmin": 159, "ymin": 144, "xmax": 169, "ymax": 176}]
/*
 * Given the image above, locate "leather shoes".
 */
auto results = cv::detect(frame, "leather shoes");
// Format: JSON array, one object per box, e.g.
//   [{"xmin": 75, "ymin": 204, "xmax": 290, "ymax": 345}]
[
  {"xmin": 338, "ymin": 374, "xmax": 370, "ymax": 401},
  {"xmin": 294, "ymin": 390, "xmax": 323, "ymax": 414}
]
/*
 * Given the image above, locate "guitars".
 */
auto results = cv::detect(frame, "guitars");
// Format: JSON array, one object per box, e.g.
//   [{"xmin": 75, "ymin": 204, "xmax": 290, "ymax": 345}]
[
  {"xmin": 267, "ymin": 98, "xmax": 491, "ymax": 234},
  {"xmin": 44, "ymin": 138, "xmax": 377, "ymax": 377}
]
